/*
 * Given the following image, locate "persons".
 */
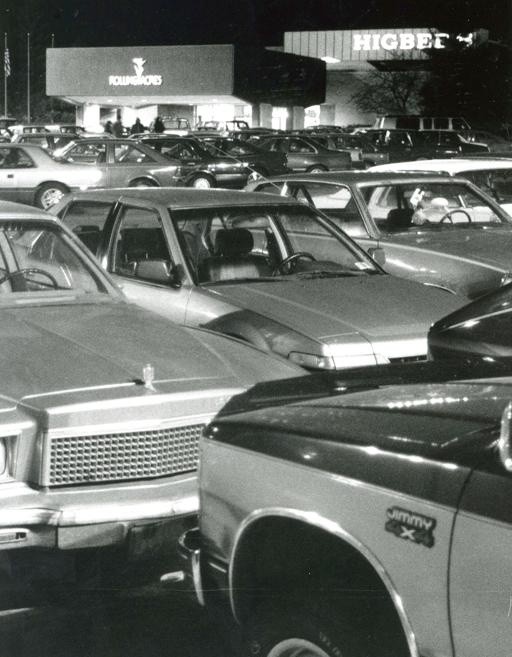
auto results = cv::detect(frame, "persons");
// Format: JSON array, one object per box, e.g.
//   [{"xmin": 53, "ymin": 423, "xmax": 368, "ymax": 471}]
[
  {"xmin": 105, "ymin": 116, "xmax": 166, "ymax": 138},
  {"xmin": 193, "ymin": 115, "xmax": 204, "ymax": 130}
]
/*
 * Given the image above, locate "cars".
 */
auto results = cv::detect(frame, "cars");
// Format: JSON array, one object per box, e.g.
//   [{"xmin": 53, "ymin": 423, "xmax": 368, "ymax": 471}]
[
  {"xmin": 44, "ymin": 186, "xmax": 474, "ymax": 370},
  {"xmin": 428, "ymin": 281, "xmax": 512, "ymax": 375},
  {"xmin": 364, "ymin": 104, "xmax": 512, "ymax": 156},
  {"xmin": 367, "ymin": 158, "xmax": 512, "ymax": 224},
  {"xmin": 241, "ymin": 173, "xmax": 512, "ymax": 300},
  {"xmin": 0, "ymin": 198, "xmax": 313, "ymax": 552},
  {"xmin": 173, "ymin": 358, "xmax": 511, "ymax": 656}
]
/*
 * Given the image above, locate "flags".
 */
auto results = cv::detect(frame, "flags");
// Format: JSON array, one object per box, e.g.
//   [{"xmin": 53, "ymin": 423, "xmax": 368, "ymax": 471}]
[{"xmin": 3, "ymin": 48, "xmax": 12, "ymax": 78}]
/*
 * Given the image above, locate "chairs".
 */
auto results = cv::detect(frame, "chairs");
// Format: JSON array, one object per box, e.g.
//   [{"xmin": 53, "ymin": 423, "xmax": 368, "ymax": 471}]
[
  {"xmin": 389, "ymin": 209, "xmax": 417, "ymax": 229},
  {"xmin": 200, "ymin": 225, "xmax": 271, "ymax": 287}
]
